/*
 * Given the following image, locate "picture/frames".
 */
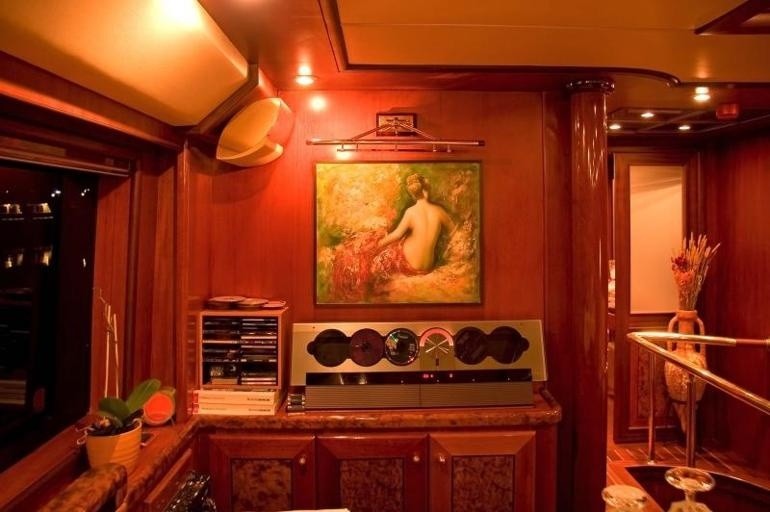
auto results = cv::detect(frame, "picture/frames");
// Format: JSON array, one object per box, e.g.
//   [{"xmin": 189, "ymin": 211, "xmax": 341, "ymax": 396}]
[{"xmin": 310, "ymin": 159, "xmax": 484, "ymax": 309}]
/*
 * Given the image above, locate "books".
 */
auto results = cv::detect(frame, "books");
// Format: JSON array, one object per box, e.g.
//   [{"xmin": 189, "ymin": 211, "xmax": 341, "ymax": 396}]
[{"xmin": 192, "ymin": 318, "xmax": 282, "ymax": 416}]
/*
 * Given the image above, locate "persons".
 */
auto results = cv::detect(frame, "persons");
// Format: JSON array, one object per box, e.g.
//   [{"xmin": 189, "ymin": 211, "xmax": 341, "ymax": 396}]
[{"xmin": 332, "ymin": 174, "xmax": 457, "ymax": 300}]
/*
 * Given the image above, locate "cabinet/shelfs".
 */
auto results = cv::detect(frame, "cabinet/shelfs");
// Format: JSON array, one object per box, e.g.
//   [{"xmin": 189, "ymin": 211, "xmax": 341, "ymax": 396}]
[
  {"xmin": 317, "ymin": 430, "xmax": 555, "ymax": 512},
  {"xmin": 127, "ymin": 423, "xmax": 204, "ymax": 512},
  {"xmin": 204, "ymin": 430, "xmax": 317, "ymax": 512}
]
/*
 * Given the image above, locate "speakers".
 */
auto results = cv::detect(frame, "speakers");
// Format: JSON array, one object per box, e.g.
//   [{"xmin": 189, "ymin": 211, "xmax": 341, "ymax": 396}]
[{"xmin": 216, "ymin": 97, "xmax": 295, "ymax": 169}]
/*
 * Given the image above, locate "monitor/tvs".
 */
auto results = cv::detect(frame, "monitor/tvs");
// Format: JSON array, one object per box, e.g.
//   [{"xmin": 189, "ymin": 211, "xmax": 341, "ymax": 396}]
[{"xmin": 0, "ymin": 159, "xmax": 101, "ymax": 476}]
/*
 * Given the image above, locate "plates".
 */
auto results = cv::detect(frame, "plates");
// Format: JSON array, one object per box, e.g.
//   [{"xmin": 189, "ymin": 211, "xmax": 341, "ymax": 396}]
[{"xmin": 206, "ymin": 295, "xmax": 287, "ymax": 311}]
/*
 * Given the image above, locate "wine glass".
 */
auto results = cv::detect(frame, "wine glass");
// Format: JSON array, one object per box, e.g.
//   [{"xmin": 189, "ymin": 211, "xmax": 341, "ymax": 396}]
[
  {"xmin": 664, "ymin": 465, "xmax": 717, "ymax": 511},
  {"xmin": 602, "ymin": 483, "xmax": 647, "ymax": 511}
]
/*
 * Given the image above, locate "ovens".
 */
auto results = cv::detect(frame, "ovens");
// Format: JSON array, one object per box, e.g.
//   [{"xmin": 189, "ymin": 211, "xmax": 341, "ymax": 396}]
[{"xmin": 163, "ymin": 472, "xmax": 217, "ymax": 510}]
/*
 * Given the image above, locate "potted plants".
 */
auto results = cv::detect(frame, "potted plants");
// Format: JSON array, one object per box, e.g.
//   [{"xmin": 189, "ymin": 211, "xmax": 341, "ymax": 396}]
[{"xmin": 80, "ymin": 378, "xmax": 161, "ymax": 476}]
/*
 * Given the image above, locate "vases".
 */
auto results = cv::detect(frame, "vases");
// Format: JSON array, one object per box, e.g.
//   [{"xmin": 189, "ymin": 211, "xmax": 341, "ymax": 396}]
[{"xmin": 663, "ymin": 310, "xmax": 709, "ymax": 439}]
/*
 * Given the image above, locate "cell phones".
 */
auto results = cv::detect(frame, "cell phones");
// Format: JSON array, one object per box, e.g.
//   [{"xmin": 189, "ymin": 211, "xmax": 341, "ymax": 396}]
[{"xmin": 140, "ymin": 432, "xmax": 156, "ymax": 447}]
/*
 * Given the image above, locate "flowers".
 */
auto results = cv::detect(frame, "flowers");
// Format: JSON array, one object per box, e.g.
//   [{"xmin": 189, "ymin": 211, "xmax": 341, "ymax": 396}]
[{"xmin": 671, "ymin": 232, "xmax": 722, "ymax": 309}]
